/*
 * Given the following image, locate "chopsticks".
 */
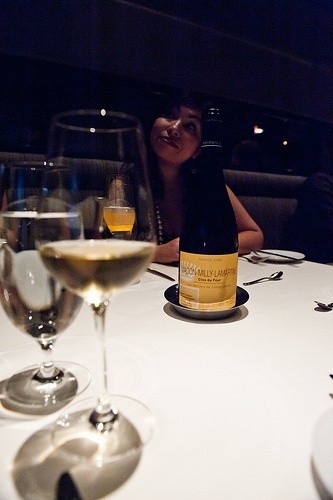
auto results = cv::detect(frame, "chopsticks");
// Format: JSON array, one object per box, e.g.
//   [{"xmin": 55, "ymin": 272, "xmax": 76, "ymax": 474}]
[{"xmin": 252, "ymin": 249, "xmax": 299, "ymax": 261}]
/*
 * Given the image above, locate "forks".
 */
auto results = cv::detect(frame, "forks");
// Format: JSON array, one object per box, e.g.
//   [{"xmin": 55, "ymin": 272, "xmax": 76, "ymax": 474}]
[{"xmin": 314, "ymin": 301, "xmax": 333, "ymax": 310}]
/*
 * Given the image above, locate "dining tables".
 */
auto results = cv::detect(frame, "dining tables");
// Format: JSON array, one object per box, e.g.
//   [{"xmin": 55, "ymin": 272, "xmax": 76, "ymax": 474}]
[{"xmin": 0, "ymin": 249, "xmax": 333, "ymax": 500}]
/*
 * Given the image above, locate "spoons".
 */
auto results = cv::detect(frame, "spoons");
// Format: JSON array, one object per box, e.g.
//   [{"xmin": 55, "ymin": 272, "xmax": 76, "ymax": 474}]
[
  {"xmin": 243, "ymin": 271, "xmax": 283, "ymax": 286},
  {"xmin": 240, "ymin": 257, "xmax": 269, "ymax": 264}
]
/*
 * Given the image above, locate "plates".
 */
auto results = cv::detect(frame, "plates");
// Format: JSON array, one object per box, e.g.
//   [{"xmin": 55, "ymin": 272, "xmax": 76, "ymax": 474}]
[
  {"xmin": 164, "ymin": 284, "xmax": 249, "ymax": 321},
  {"xmin": 250, "ymin": 249, "xmax": 306, "ymax": 263}
]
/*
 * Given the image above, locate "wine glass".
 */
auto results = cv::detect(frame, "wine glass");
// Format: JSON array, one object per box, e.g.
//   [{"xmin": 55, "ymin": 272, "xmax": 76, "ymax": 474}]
[
  {"xmin": 102, "ymin": 174, "xmax": 140, "ymax": 286},
  {"xmin": 0, "ymin": 161, "xmax": 90, "ymax": 405},
  {"xmin": 34, "ymin": 109, "xmax": 157, "ymax": 465}
]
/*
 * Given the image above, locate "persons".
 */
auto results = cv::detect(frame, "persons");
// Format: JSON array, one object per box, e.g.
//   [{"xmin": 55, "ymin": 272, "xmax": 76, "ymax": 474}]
[{"xmin": 101, "ymin": 86, "xmax": 265, "ymax": 265}]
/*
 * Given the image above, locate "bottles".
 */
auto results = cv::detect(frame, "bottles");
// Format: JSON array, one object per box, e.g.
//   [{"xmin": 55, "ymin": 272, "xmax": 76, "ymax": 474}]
[{"xmin": 177, "ymin": 108, "xmax": 238, "ymax": 312}]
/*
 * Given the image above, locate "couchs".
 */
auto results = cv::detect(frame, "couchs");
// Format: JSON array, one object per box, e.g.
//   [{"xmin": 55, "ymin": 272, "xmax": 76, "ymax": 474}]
[{"xmin": 0, "ymin": 151, "xmax": 309, "ymax": 249}]
